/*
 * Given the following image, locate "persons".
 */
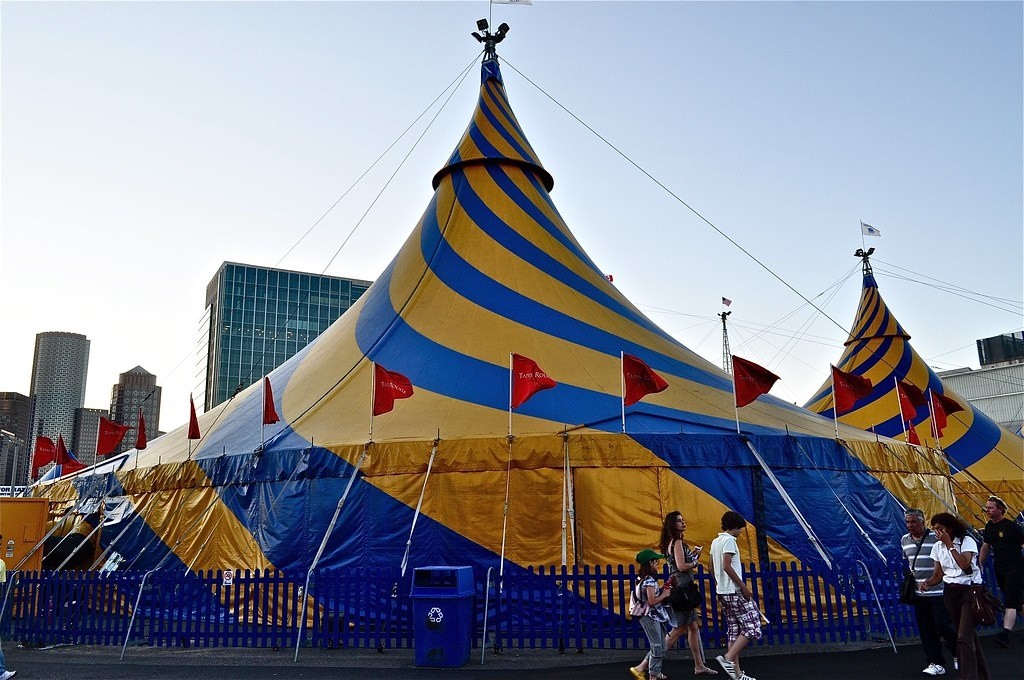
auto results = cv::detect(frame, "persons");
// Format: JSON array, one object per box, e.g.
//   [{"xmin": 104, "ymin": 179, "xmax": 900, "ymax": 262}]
[
  {"xmin": 0, "ymin": 534, "xmax": 16, "ymax": 680},
  {"xmin": 628, "ymin": 511, "xmax": 718, "ymax": 680},
  {"xmin": 901, "ymin": 496, "xmax": 1024, "ymax": 680},
  {"xmin": 708, "ymin": 511, "xmax": 762, "ymax": 680}
]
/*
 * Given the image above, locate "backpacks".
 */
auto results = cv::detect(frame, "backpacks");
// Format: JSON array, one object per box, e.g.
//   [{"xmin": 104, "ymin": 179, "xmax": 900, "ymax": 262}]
[{"xmin": 629, "ymin": 575, "xmax": 657, "ymax": 617}]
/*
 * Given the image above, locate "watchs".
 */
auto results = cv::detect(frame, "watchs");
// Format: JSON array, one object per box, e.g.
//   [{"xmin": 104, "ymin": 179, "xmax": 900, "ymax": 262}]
[
  {"xmin": 950, "ymin": 547, "xmax": 955, "ymax": 551},
  {"xmin": 693, "ymin": 562, "xmax": 697, "ymax": 568}
]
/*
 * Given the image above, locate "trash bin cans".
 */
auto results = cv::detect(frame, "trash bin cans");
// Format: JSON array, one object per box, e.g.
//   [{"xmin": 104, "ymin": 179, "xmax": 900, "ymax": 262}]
[{"xmin": 409, "ymin": 565, "xmax": 475, "ymax": 667}]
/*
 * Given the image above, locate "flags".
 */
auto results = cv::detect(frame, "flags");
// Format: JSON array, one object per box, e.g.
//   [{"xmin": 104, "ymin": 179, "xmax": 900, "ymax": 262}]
[
  {"xmin": 374, "ymin": 363, "xmax": 414, "ymax": 416},
  {"xmin": 264, "ymin": 377, "xmax": 280, "ymax": 424},
  {"xmin": 623, "ymin": 353, "xmax": 669, "ymax": 407},
  {"xmin": 909, "ymin": 422, "xmax": 921, "ymax": 446},
  {"xmin": 512, "ymin": 353, "xmax": 557, "ymax": 409},
  {"xmin": 833, "ymin": 366, "xmax": 874, "ymax": 414},
  {"xmin": 136, "ymin": 414, "xmax": 149, "ymax": 450},
  {"xmin": 605, "ymin": 275, "xmax": 613, "ymax": 282},
  {"xmin": 96, "ymin": 416, "xmax": 135, "ymax": 455},
  {"xmin": 862, "ymin": 223, "xmax": 881, "ymax": 237},
  {"xmin": 929, "ymin": 392, "xmax": 965, "ymax": 438},
  {"xmin": 897, "ymin": 382, "xmax": 927, "ymax": 422},
  {"xmin": 188, "ymin": 399, "xmax": 201, "ymax": 439},
  {"xmin": 31, "ymin": 433, "xmax": 70, "ymax": 476},
  {"xmin": 732, "ymin": 355, "xmax": 781, "ymax": 408},
  {"xmin": 723, "ymin": 297, "xmax": 732, "ymax": 306}
]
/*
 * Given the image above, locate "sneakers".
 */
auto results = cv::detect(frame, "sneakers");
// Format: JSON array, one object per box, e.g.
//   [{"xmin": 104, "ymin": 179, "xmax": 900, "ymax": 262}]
[
  {"xmin": 728, "ymin": 672, "xmax": 756, "ymax": 680},
  {"xmin": 953, "ymin": 656, "xmax": 959, "ymax": 670},
  {"xmin": 0, "ymin": 670, "xmax": 16, "ymax": 680},
  {"xmin": 921, "ymin": 663, "xmax": 946, "ymax": 676},
  {"xmin": 714, "ymin": 654, "xmax": 737, "ymax": 680}
]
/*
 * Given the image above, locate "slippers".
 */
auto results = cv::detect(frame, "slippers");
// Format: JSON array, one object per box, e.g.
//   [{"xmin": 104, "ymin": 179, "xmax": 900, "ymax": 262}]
[{"xmin": 629, "ymin": 667, "xmax": 646, "ymax": 680}]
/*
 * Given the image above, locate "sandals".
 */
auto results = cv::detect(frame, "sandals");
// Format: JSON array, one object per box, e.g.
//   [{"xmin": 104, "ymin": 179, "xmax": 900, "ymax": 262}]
[
  {"xmin": 694, "ymin": 668, "xmax": 719, "ymax": 676},
  {"xmin": 649, "ymin": 672, "xmax": 667, "ymax": 680}
]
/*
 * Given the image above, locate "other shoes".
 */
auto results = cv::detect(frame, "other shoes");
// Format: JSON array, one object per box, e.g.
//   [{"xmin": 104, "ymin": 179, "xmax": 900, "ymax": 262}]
[{"xmin": 992, "ymin": 634, "xmax": 1009, "ymax": 648}]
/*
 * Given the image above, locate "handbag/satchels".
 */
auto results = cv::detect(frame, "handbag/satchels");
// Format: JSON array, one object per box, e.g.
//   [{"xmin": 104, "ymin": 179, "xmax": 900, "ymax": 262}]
[
  {"xmin": 899, "ymin": 570, "xmax": 917, "ymax": 605},
  {"xmin": 968, "ymin": 581, "xmax": 1006, "ymax": 626},
  {"xmin": 670, "ymin": 581, "xmax": 703, "ymax": 612}
]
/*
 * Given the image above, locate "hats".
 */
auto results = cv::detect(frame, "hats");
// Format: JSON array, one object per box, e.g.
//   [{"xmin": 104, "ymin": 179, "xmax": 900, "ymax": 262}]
[{"xmin": 636, "ymin": 549, "xmax": 663, "ymax": 564}]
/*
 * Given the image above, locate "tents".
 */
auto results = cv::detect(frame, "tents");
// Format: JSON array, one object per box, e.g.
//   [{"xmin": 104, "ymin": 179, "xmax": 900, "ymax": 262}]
[
  {"xmin": 16, "ymin": 449, "xmax": 80, "ymax": 497},
  {"xmin": 15, "ymin": 54, "xmax": 961, "ymax": 639},
  {"xmin": 801, "ymin": 272, "xmax": 1024, "ymax": 530}
]
